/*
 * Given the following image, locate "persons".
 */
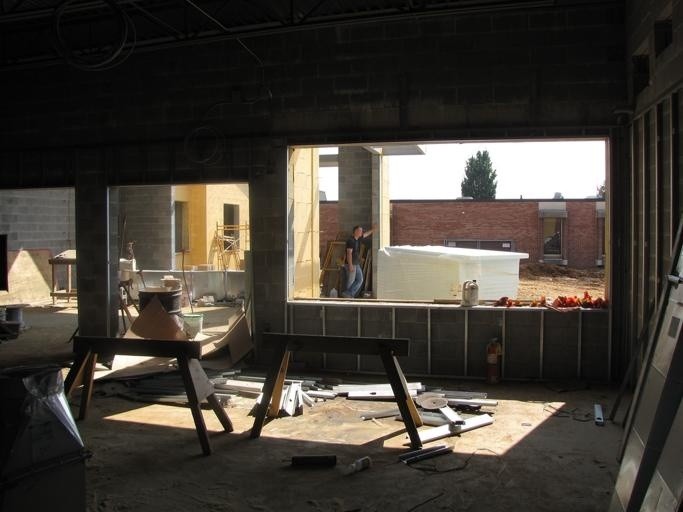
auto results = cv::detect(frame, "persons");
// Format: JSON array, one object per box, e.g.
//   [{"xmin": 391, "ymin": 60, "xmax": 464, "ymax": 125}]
[{"xmin": 341, "ymin": 222, "xmax": 378, "ymax": 298}]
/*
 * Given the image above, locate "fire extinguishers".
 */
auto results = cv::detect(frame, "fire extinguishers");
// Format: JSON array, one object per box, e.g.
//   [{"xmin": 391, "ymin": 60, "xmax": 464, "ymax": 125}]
[{"xmin": 485, "ymin": 336, "xmax": 501, "ymax": 363}]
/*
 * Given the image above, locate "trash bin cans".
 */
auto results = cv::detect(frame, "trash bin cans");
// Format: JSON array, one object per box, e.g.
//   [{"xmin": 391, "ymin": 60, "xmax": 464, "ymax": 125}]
[{"xmin": 183, "ymin": 313, "xmax": 203, "ymax": 340}]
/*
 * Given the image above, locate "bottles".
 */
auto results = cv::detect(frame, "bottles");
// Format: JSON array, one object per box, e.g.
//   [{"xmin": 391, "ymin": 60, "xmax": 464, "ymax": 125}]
[
  {"xmin": 471, "ymin": 279, "xmax": 479, "ymax": 306},
  {"xmin": 350, "ymin": 455, "xmax": 371, "ymax": 472}
]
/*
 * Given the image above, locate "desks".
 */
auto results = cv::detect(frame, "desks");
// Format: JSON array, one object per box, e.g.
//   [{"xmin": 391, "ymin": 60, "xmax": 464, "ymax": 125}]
[{"xmin": 49, "ymin": 258, "xmax": 77, "ymax": 305}]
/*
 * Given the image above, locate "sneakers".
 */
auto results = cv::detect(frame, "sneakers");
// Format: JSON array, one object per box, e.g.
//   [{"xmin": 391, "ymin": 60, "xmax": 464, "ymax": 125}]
[{"xmin": 342, "ymin": 292, "xmax": 352, "ymax": 298}]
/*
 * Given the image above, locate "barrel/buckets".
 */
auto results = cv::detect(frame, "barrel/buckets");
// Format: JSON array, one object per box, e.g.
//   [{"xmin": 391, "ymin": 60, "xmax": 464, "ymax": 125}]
[
  {"xmin": 139, "ymin": 289, "xmax": 181, "ymax": 324},
  {"xmin": 461, "ymin": 279, "xmax": 478, "ymax": 306}
]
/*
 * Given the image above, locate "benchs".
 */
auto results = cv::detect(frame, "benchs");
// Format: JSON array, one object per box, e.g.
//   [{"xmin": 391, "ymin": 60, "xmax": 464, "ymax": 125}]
[
  {"xmin": 249, "ymin": 330, "xmax": 421, "ymax": 450},
  {"xmin": 64, "ymin": 337, "xmax": 233, "ymax": 455}
]
